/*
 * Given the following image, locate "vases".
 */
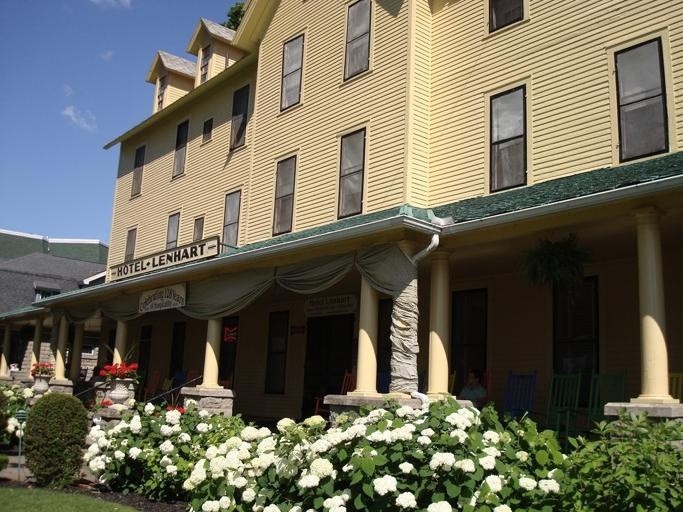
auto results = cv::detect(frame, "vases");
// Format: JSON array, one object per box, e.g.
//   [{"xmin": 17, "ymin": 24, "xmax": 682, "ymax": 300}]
[
  {"xmin": 32, "ymin": 374, "xmax": 52, "ymax": 393},
  {"xmin": 108, "ymin": 378, "xmax": 137, "ymax": 402}
]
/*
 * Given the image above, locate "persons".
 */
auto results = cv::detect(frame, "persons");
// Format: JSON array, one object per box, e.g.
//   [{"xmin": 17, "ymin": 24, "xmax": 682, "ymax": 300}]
[{"xmin": 456, "ymin": 367, "xmax": 488, "ymax": 408}]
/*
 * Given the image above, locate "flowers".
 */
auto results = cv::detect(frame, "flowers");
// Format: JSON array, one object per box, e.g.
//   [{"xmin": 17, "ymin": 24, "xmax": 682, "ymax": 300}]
[
  {"xmin": 31, "ymin": 363, "xmax": 55, "ymax": 376},
  {"xmin": 99, "ymin": 363, "xmax": 144, "ymax": 387}
]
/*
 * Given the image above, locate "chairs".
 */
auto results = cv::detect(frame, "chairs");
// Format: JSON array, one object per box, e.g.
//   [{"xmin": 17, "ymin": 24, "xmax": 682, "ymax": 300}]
[{"xmin": 144, "ymin": 369, "xmax": 238, "ymax": 413}]
